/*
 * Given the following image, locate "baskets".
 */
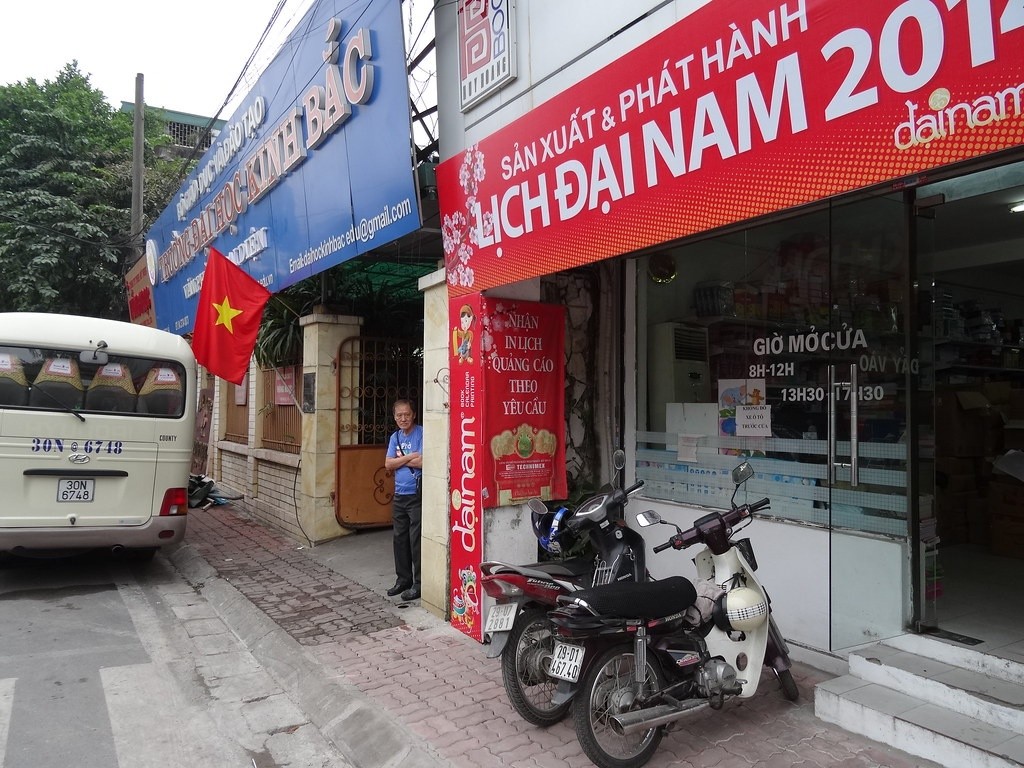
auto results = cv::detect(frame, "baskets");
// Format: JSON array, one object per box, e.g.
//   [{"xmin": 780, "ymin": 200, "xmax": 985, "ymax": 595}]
[
  {"xmin": 571, "ymin": 539, "xmax": 592, "ymax": 555},
  {"xmin": 691, "ymin": 538, "xmax": 758, "ymax": 572}
]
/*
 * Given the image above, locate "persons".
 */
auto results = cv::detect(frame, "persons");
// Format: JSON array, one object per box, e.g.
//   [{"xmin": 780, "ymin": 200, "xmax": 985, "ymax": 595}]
[{"xmin": 384, "ymin": 399, "xmax": 423, "ymax": 601}]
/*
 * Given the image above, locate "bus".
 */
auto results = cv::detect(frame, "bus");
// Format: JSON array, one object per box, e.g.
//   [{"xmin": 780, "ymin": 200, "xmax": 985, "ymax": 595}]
[{"xmin": 0, "ymin": 311, "xmax": 198, "ymax": 563}]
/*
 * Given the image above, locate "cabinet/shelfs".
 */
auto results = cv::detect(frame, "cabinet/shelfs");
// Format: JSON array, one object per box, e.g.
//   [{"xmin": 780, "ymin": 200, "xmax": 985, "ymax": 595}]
[{"xmin": 704, "ymin": 317, "xmax": 1024, "ymax": 442}]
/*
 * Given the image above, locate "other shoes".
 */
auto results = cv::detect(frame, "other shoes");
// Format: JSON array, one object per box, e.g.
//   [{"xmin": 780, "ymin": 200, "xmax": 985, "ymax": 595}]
[
  {"xmin": 401, "ymin": 588, "xmax": 421, "ymax": 601},
  {"xmin": 387, "ymin": 582, "xmax": 412, "ymax": 596}
]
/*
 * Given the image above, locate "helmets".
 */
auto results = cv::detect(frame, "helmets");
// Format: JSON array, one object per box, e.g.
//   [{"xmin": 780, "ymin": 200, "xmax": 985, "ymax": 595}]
[
  {"xmin": 531, "ymin": 503, "xmax": 579, "ymax": 554},
  {"xmin": 712, "ymin": 587, "xmax": 766, "ymax": 631}
]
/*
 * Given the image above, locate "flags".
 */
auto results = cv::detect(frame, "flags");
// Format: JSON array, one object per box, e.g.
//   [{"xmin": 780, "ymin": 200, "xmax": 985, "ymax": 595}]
[{"xmin": 191, "ymin": 246, "xmax": 270, "ymax": 386}]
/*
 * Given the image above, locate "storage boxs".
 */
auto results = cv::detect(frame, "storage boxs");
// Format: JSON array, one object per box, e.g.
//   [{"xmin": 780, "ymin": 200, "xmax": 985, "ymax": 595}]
[
  {"xmin": 698, "ymin": 281, "xmax": 734, "ymax": 315},
  {"xmin": 935, "ymin": 388, "xmax": 1024, "ymax": 559}
]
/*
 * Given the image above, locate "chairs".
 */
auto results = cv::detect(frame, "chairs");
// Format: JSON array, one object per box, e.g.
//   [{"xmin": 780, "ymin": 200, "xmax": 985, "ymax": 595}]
[{"xmin": 0, "ymin": 351, "xmax": 182, "ymax": 417}]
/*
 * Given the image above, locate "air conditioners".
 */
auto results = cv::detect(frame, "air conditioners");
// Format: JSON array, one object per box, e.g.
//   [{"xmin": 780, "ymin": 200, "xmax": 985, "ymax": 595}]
[{"xmin": 647, "ymin": 322, "xmax": 711, "ymax": 450}]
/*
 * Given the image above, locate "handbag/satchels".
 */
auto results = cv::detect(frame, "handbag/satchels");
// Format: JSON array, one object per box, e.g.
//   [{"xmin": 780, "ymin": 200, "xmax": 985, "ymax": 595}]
[{"xmin": 416, "ymin": 476, "xmax": 422, "ymax": 500}]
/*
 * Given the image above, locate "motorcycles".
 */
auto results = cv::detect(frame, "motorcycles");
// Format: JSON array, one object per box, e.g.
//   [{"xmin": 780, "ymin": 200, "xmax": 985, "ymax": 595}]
[
  {"xmin": 545, "ymin": 461, "xmax": 801, "ymax": 768},
  {"xmin": 479, "ymin": 447, "xmax": 653, "ymax": 729}
]
